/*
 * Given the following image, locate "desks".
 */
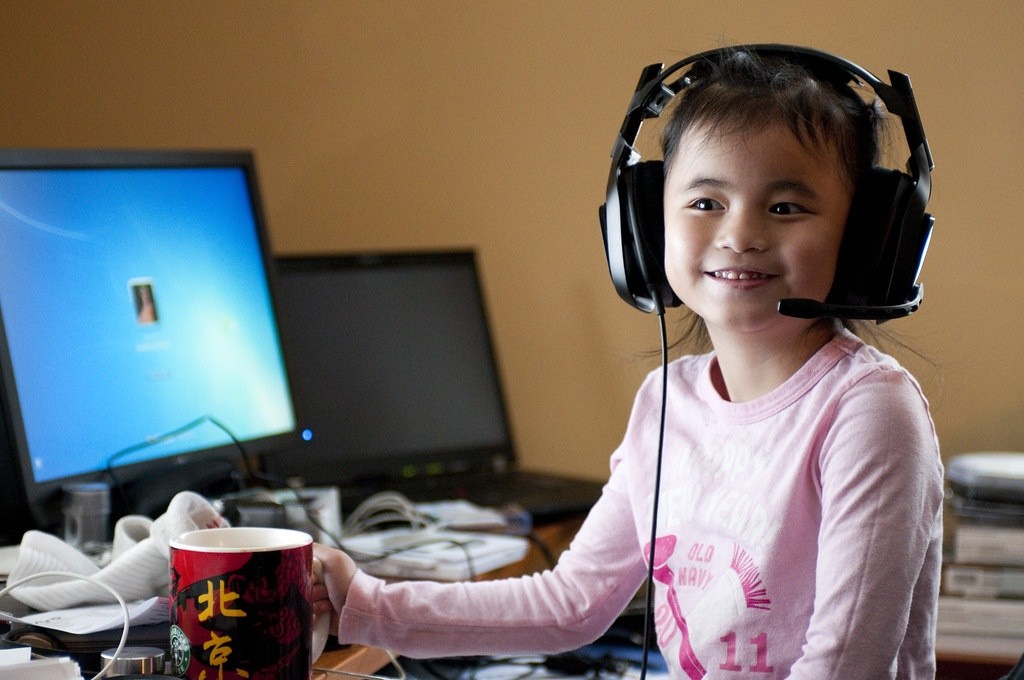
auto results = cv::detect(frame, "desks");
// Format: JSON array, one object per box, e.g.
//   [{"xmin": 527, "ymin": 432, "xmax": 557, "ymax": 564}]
[{"xmin": 308, "ymin": 518, "xmax": 577, "ymax": 680}]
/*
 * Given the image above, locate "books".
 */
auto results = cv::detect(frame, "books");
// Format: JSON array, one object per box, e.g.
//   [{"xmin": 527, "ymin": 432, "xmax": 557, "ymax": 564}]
[{"xmin": 931, "ymin": 448, "xmax": 1024, "ymax": 659}]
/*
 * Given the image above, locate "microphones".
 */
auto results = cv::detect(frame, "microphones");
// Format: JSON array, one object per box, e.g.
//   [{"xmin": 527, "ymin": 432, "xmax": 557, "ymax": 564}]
[{"xmin": 776, "ymin": 282, "xmax": 924, "ymax": 320}]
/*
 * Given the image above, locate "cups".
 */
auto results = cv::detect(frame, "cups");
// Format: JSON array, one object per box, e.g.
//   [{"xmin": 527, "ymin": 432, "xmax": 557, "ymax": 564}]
[{"xmin": 168, "ymin": 527, "xmax": 333, "ymax": 680}]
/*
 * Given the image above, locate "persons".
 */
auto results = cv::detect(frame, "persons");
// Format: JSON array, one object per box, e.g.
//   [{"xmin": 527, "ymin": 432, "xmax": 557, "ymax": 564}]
[{"xmin": 311, "ymin": 43, "xmax": 948, "ymax": 680}]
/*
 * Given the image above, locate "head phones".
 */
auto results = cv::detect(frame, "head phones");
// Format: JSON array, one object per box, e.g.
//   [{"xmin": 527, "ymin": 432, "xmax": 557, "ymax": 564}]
[{"xmin": 597, "ymin": 43, "xmax": 935, "ymax": 322}]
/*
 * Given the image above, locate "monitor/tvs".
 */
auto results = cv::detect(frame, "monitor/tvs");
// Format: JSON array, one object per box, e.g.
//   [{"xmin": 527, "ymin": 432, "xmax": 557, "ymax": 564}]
[
  {"xmin": 0, "ymin": 145, "xmax": 312, "ymax": 506},
  {"xmin": 262, "ymin": 246, "xmax": 519, "ymax": 487}
]
